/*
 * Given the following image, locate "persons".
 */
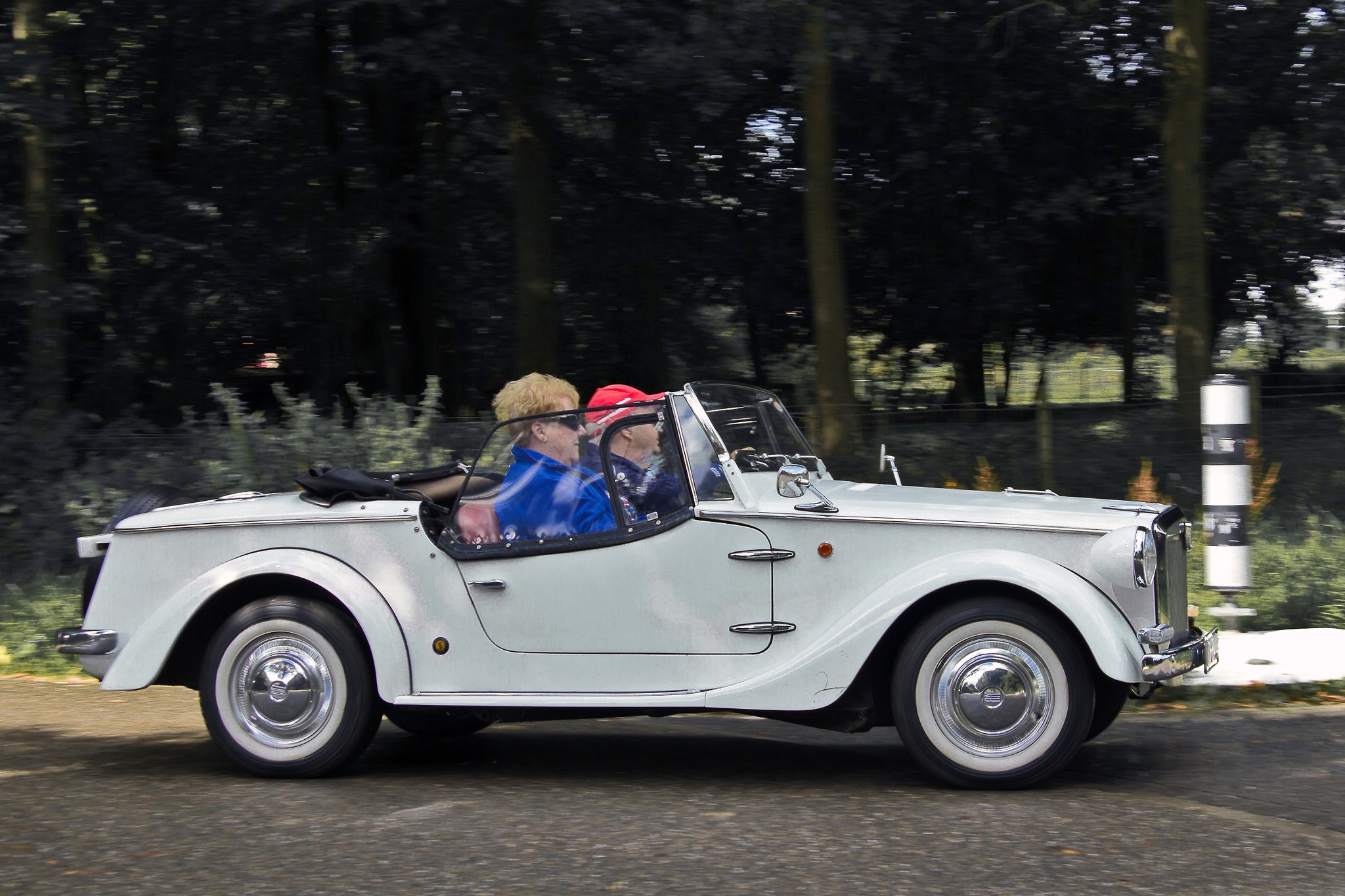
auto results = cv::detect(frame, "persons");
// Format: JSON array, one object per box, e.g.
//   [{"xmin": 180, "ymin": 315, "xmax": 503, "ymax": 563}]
[
  {"xmin": 491, "ymin": 373, "xmax": 641, "ymax": 541},
  {"xmin": 584, "ymin": 383, "xmax": 756, "ymax": 515}
]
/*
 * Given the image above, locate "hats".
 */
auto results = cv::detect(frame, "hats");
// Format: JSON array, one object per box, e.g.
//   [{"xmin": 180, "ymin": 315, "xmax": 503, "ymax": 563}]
[{"xmin": 585, "ymin": 384, "xmax": 665, "ymax": 438}]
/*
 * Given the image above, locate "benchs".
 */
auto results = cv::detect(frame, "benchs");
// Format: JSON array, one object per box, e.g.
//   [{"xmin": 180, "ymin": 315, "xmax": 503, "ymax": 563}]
[{"xmin": 457, "ymin": 486, "xmax": 501, "ymax": 549}]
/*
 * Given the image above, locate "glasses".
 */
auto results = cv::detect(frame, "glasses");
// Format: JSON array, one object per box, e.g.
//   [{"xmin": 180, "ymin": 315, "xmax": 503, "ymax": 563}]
[{"xmin": 544, "ymin": 414, "xmax": 580, "ymax": 430}]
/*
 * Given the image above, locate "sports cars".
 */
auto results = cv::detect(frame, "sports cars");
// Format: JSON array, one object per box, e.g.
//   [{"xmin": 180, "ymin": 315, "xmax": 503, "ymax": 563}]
[{"xmin": 55, "ymin": 379, "xmax": 1219, "ymax": 789}]
